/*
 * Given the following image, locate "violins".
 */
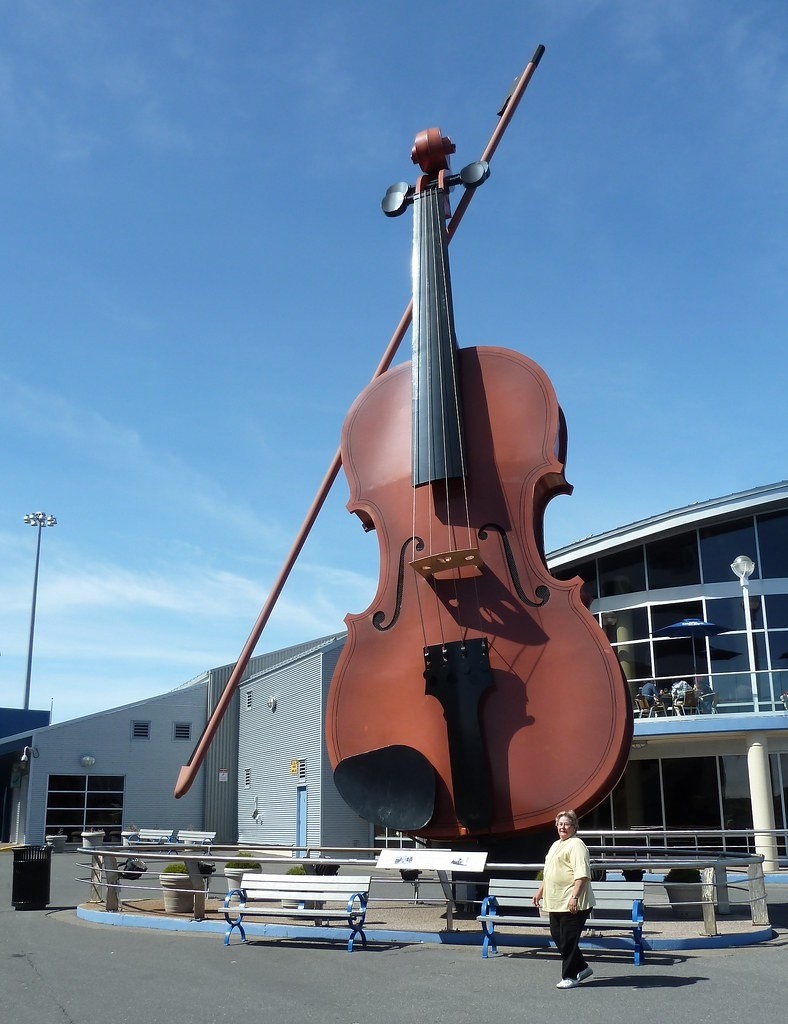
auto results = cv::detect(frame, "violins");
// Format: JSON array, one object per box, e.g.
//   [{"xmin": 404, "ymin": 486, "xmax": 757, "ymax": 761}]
[{"xmin": 329, "ymin": 121, "xmax": 634, "ymax": 844}]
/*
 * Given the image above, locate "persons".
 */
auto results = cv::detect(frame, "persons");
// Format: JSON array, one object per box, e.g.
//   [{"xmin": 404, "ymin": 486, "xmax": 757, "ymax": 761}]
[
  {"xmin": 637, "ymin": 676, "xmax": 692, "ymax": 716},
  {"xmin": 532, "ymin": 810, "xmax": 596, "ymax": 989},
  {"xmin": 692, "ymin": 676, "xmax": 714, "ymax": 711},
  {"xmin": 153, "ymin": 824, "xmax": 160, "ymax": 830}
]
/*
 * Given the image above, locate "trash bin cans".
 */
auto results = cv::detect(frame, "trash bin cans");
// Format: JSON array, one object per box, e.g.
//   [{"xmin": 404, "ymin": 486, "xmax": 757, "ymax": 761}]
[{"xmin": 11, "ymin": 846, "xmax": 55, "ymax": 910}]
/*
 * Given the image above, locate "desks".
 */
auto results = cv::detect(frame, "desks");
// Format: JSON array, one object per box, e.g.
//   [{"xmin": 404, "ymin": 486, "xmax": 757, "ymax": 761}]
[{"xmin": 660, "ymin": 695, "xmax": 672, "ymax": 703}]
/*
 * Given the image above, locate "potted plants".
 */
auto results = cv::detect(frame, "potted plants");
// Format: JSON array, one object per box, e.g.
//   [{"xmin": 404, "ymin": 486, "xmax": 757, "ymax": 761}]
[
  {"xmin": 224, "ymin": 851, "xmax": 263, "ymax": 902},
  {"xmin": 159, "ymin": 863, "xmax": 194, "ymax": 913},
  {"xmin": 121, "ymin": 824, "xmax": 140, "ymax": 846},
  {"xmin": 664, "ymin": 870, "xmax": 703, "ymax": 918},
  {"xmin": 280, "ymin": 866, "xmax": 315, "ymax": 908},
  {"xmin": 81, "ymin": 828, "xmax": 106, "ymax": 848},
  {"xmin": 46, "ymin": 828, "xmax": 68, "ymax": 852}
]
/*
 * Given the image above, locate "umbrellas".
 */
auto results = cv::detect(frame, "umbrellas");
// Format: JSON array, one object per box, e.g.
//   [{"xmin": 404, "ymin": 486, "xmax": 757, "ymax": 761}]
[{"xmin": 648, "ymin": 619, "xmax": 731, "ymax": 685}]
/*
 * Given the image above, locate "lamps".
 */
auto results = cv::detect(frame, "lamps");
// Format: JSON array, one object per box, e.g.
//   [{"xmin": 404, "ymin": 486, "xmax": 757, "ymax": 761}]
[{"xmin": 117, "ymin": 857, "xmax": 148, "ymax": 880}]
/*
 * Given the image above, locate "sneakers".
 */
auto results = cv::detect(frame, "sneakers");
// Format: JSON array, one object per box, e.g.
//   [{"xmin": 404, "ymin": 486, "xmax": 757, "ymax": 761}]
[
  {"xmin": 556, "ymin": 977, "xmax": 579, "ymax": 988},
  {"xmin": 576, "ymin": 965, "xmax": 593, "ymax": 981}
]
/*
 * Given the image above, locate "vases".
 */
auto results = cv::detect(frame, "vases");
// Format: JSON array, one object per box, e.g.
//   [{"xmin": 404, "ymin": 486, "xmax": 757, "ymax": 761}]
[
  {"xmin": 71, "ymin": 831, "xmax": 82, "ymax": 842},
  {"xmin": 109, "ymin": 831, "xmax": 121, "ymax": 842}
]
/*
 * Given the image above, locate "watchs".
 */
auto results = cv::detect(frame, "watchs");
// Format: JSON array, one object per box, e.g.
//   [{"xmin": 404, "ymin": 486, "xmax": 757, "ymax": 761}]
[{"xmin": 571, "ymin": 895, "xmax": 579, "ymax": 899}]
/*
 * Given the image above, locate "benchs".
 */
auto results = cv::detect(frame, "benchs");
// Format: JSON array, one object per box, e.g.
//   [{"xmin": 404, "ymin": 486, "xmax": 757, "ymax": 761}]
[
  {"xmin": 126, "ymin": 829, "xmax": 174, "ymax": 854},
  {"xmin": 218, "ymin": 873, "xmax": 372, "ymax": 952},
  {"xmin": 164, "ymin": 830, "xmax": 217, "ymax": 856},
  {"xmin": 476, "ymin": 878, "xmax": 645, "ymax": 966}
]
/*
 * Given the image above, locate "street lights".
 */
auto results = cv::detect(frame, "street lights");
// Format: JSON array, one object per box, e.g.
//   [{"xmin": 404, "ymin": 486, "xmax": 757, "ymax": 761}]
[
  {"xmin": 24, "ymin": 512, "xmax": 57, "ymax": 710},
  {"xmin": 730, "ymin": 555, "xmax": 759, "ymax": 712}
]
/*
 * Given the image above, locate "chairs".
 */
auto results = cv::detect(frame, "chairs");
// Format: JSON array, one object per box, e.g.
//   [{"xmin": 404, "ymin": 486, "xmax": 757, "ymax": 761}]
[{"xmin": 635, "ymin": 690, "xmax": 720, "ymax": 718}]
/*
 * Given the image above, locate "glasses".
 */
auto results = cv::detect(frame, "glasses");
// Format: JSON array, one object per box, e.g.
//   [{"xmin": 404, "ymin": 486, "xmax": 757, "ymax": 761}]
[{"xmin": 555, "ymin": 821, "xmax": 575, "ymax": 828}]
